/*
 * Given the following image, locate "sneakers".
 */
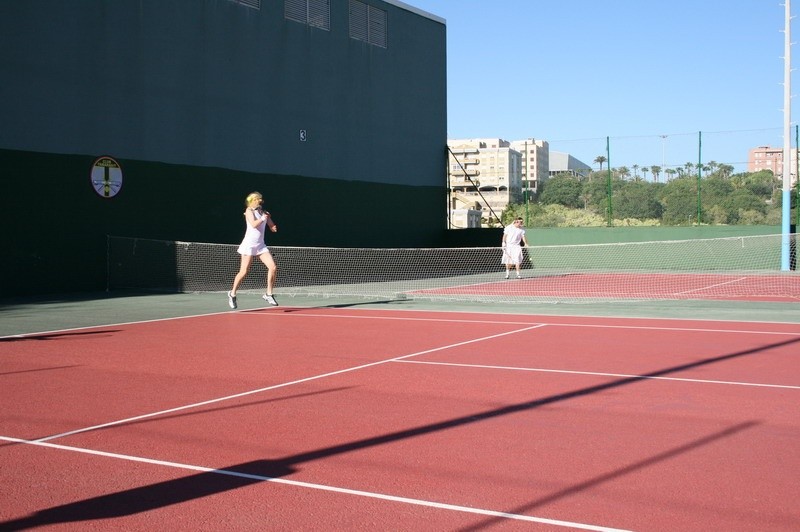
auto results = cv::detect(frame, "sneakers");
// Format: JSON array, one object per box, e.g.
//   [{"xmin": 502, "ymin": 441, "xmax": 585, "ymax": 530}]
[
  {"xmin": 227, "ymin": 290, "xmax": 237, "ymax": 309},
  {"xmin": 263, "ymin": 294, "xmax": 279, "ymax": 306}
]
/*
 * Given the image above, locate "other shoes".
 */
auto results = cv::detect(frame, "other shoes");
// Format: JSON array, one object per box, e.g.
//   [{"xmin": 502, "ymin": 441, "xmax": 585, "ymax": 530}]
[
  {"xmin": 506, "ymin": 274, "xmax": 510, "ymax": 279},
  {"xmin": 516, "ymin": 275, "xmax": 522, "ymax": 279}
]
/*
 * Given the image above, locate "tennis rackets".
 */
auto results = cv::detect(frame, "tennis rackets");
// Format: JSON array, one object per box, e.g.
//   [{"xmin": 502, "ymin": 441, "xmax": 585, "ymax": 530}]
[{"xmin": 503, "ymin": 248, "xmax": 521, "ymax": 263}]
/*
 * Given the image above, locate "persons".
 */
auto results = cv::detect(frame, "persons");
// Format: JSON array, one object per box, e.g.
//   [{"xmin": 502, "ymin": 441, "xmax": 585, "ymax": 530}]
[
  {"xmin": 228, "ymin": 192, "xmax": 278, "ymax": 309},
  {"xmin": 501, "ymin": 217, "xmax": 527, "ymax": 279}
]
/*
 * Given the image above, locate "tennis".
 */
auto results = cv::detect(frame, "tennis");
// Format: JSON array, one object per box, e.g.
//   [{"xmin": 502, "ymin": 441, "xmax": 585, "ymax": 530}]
[{"xmin": 529, "ymin": 257, "xmax": 532, "ymax": 260}]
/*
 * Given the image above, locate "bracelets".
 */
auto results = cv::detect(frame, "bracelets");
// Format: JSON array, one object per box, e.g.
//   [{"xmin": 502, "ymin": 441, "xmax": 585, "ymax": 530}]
[{"xmin": 261, "ymin": 216, "xmax": 266, "ymax": 222}]
